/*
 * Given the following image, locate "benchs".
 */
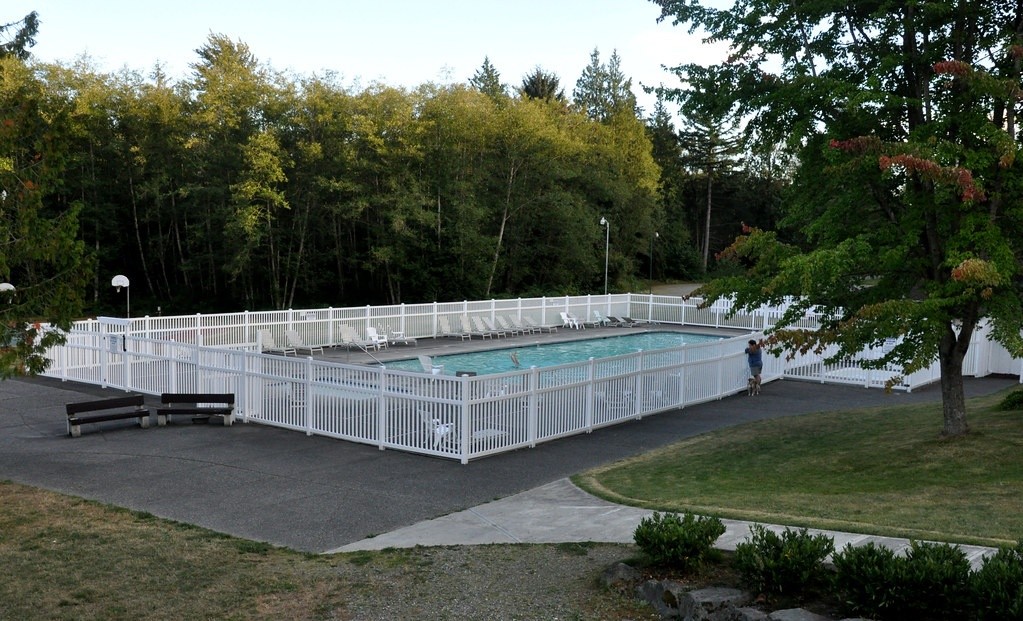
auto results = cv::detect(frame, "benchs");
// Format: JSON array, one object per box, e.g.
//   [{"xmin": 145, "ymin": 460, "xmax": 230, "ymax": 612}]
[
  {"xmin": 66, "ymin": 393, "xmax": 150, "ymax": 437},
  {"xmin": 157, "ymin": 392, "xmax": 235, "ymax": 428}
]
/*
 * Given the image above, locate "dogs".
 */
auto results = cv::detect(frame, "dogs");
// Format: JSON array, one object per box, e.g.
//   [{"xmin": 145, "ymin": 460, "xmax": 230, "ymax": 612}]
[{"xmin": 747, "ymin": 377, "xmax": 760, "ymax": 396}]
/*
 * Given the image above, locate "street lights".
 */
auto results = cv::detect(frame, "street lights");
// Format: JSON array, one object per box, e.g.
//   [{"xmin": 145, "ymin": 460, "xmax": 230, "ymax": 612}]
[
  {"xmin": 600, "ymin": 218, "xmax": 609, "ymax": 293},
  {"xmin": 649, "ymin": 232, "xmax": 659, "ymax": 294}
]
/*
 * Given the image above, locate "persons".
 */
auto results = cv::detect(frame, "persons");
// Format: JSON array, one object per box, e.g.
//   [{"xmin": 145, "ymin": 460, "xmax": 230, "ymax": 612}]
[{"xmin": 745, "ymin": 340, "xmax": 763, "ymax": 391}]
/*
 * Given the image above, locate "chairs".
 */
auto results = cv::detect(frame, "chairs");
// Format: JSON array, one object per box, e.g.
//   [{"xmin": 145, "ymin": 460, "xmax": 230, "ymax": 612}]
[
  {"xmin": 417, "ymin": 408, "xmax": 510, "ymax": 453},
  {"xmin": 559, "ymin": 311, "xmax": 660, "ymax": 330},
  {"xmin": 287, "ymin": 373, "xmax": 307, "ymax": 408},
  {"xmin": 418, "ymin": 355, "xmax": 444, "ymax": 382},
  {"xmin": 595, "ymin": 370, "xmax": 673, "ymax": 413},
  {"xmin": 333, "ymin": 322, "xmax": 418, "ymax": 354},
  {"xmin": 258, "ymin": 328, "xmax": 297, "ymax": 357},
  {"xmin": 285, "ymin": 329, "xmax": 324, "ymax": 356},
  {"xmin": 484, "ymin": 374, "xmax": 544, "ymax": 409},
  {"xmin": 439, "ymin": 315, "xmax": 558, "ymax": 341}
]
